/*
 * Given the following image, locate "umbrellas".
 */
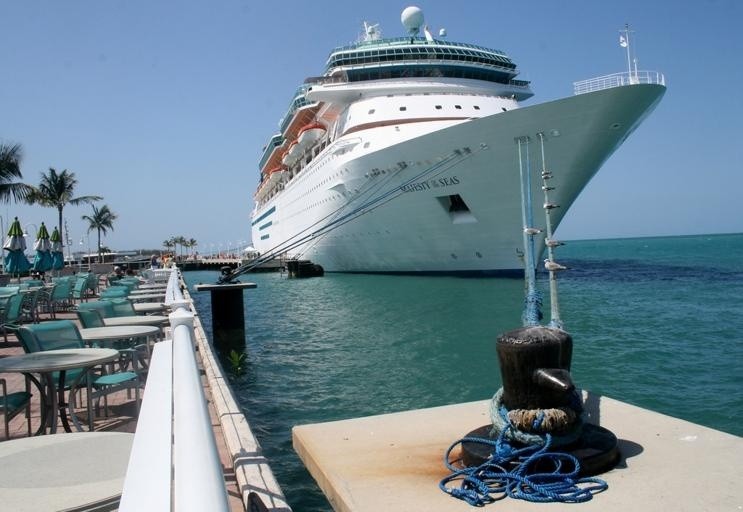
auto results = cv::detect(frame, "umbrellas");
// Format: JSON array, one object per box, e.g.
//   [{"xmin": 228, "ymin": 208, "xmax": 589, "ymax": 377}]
[
  {"xmin": 30, "ymin": 221, "xmax": 52, "ymax": 280},
  {"xmin": 48, "ymin": 225, "xmax": 65, "ymax": 280},
  {"xmin": 1, "ymin": 216, "xmax": 31, "ymax": 284}
]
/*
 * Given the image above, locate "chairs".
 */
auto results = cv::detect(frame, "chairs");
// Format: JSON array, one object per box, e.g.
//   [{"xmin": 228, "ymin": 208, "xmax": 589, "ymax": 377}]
[{"xmin": 0, "ymin": 272, "xmax": 142, "ymax": 441}]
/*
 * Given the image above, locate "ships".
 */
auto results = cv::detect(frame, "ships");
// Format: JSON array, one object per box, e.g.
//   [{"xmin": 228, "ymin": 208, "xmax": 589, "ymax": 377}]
[{"xmin": 250, "ymin": 5, "xmax": 667, "ymax": 278}]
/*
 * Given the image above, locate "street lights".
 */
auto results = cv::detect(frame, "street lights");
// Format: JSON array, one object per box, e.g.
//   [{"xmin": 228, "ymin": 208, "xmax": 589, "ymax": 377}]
[
  {"xmin": 79, "ymin": 234, "xmax": 92, "ymax": 273},
  {"xmin": 23, "ymin": 222, "xmax": 39, "ymax": 242}
]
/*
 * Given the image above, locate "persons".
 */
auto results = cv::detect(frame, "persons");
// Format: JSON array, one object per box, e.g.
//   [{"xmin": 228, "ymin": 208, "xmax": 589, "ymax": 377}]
[
  {"xmin": 150, "ymin": 254, "xmax": 174, "ymax": 269},
  {"xmin": 108, "ymin": 265, "xmax": 125, "ymax": 277},
  {"xmin": 124, "ymin": 268, "xmax": 138, "ymax": 275}
]
